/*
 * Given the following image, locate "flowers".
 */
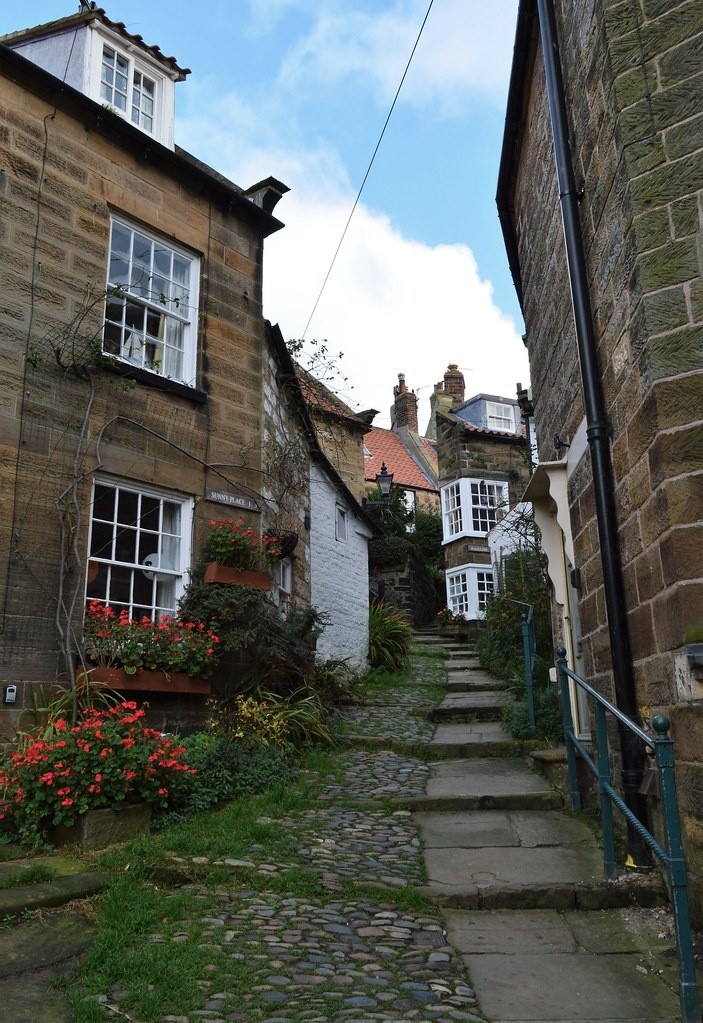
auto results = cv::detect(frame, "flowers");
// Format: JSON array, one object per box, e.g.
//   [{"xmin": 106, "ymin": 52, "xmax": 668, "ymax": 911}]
[
  {"xmin": 0, "ymin": 700, "xmax": 200, "ymax": 821},
  {"xmin": 192, "ymin": 512, "xmax": 282, "ymax": 564},
  {"xmin": 436, "ymin": 605, "xmax": 465, "ymax": 624},
  {"xmin": 80, "ymin": 599, "xmax": 220, "ymax": 676}
]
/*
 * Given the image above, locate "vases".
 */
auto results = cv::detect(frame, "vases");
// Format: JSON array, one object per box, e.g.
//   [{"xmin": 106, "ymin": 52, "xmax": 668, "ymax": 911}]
[
  {"xmin": 443, "ymin": 625, "xmax": 462, "ymax": 630},
  {"xmin": 75, "ymin": 665, "xmax": 212, "ymax": 693},
  {"xmin": 203, "ymin": 563, "xmax": 270, "ymax": 592},
  {"xmin": 59, "ymin": 802, "xmax": 152, "ymax": 850}
]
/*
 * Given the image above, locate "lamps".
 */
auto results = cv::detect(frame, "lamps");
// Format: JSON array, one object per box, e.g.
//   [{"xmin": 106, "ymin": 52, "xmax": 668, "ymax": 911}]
[{"xmin": 362, "ymin": 461, "xmax": 394, "ymax": 506}]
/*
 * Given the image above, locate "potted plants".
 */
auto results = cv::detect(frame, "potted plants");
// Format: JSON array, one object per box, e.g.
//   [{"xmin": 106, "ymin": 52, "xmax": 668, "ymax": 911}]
[{"xmin": 268, "ymin": 511, "xmax": 302, "ymax": 560}]
